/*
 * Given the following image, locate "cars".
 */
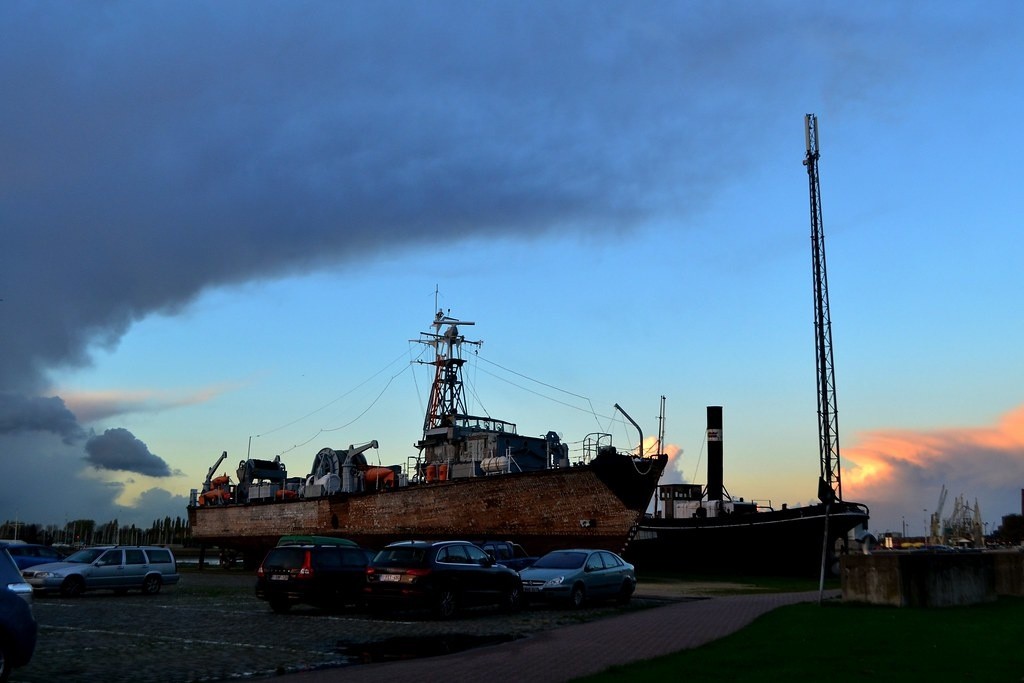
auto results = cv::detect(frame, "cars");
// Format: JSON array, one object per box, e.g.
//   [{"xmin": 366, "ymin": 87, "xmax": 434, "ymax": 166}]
[
  {"xmin": 515, "ymin": 547, "xmax": 638, "ymax": 610},
  {"xmin": 4, "ymin": 542, "xmax": 84, "ymax": 572},
  {"xmin": 18, "ymin": 544, "xmax": 181, "ymax": 600},
  {"xmin": 0, "ymin": 540, "xmax": 38, "ymax": 683}
]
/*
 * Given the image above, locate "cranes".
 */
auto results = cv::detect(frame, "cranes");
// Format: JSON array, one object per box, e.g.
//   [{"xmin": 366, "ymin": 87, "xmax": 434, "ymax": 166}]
[{"xmin": 854, "ymin": 485, "xmax": 989, "ymax": 555}]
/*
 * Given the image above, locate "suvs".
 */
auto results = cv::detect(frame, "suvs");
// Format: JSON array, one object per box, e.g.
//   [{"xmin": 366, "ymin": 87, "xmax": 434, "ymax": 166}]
[
  {"xmin": 466, "ymin": 539, "xmax": 535, "ymax": 573},
  {"xmin": 360, "ymin": 538, "xmax": 522, "ymax": 620},
  {"xmin": 255, "ymin": 542, "xmax": 378, "ymax": 612}
]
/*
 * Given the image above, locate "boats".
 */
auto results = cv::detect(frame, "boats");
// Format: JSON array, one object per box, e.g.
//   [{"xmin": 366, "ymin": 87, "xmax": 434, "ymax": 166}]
[
  {"xmin": 187, "ymin": 308, "xmax": 671, "ymax": 560},
  {"xmin": 611, "ymin": 395, "xmax": 871, "ymax": 578}
]
